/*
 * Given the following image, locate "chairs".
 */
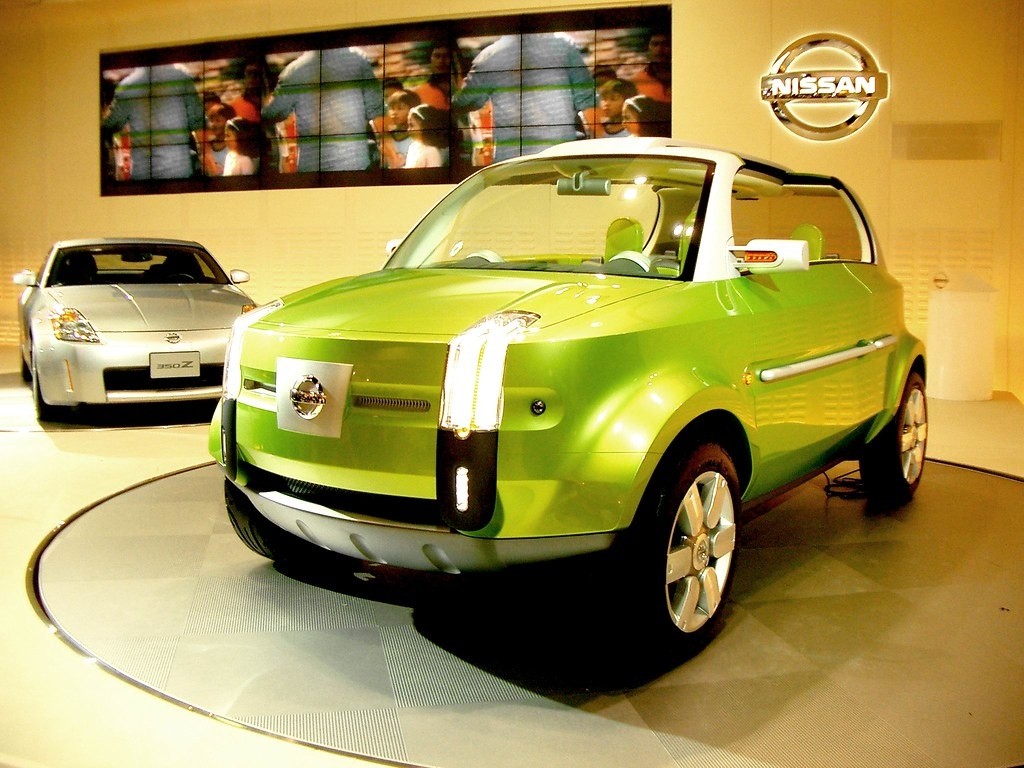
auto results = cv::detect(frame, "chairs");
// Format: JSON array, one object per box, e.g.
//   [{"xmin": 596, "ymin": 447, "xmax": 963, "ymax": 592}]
[
  {"xmin": 604, "ymin": 209, "xmax": 826, "ymax": 278},
  {"xmin": 67, "ymin": 252, "xmax": 201, "ymax": 285}
]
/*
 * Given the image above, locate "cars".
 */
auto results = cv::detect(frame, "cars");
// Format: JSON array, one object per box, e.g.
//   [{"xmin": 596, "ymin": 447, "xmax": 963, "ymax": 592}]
[
  {"xmin": 12, "ymin": 238, "xmax": 258, "ymax": 422},
  {"xmin": 205, "ymin": 136, "xmax": 927, "ymax": 651}
]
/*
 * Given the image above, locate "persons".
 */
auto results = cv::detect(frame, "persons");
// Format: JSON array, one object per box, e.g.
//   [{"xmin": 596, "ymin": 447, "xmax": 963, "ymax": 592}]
[
  {"xmin": 452, "ymin": 32, "xmax": 671, "ymax": 161},
  {"xmin": 102, "ymin": 63, "xmax": 261, "ymax": 179},
  {"xmin": 261, "ymin": 39, "xmax": 451, "ymax": 167}
]
